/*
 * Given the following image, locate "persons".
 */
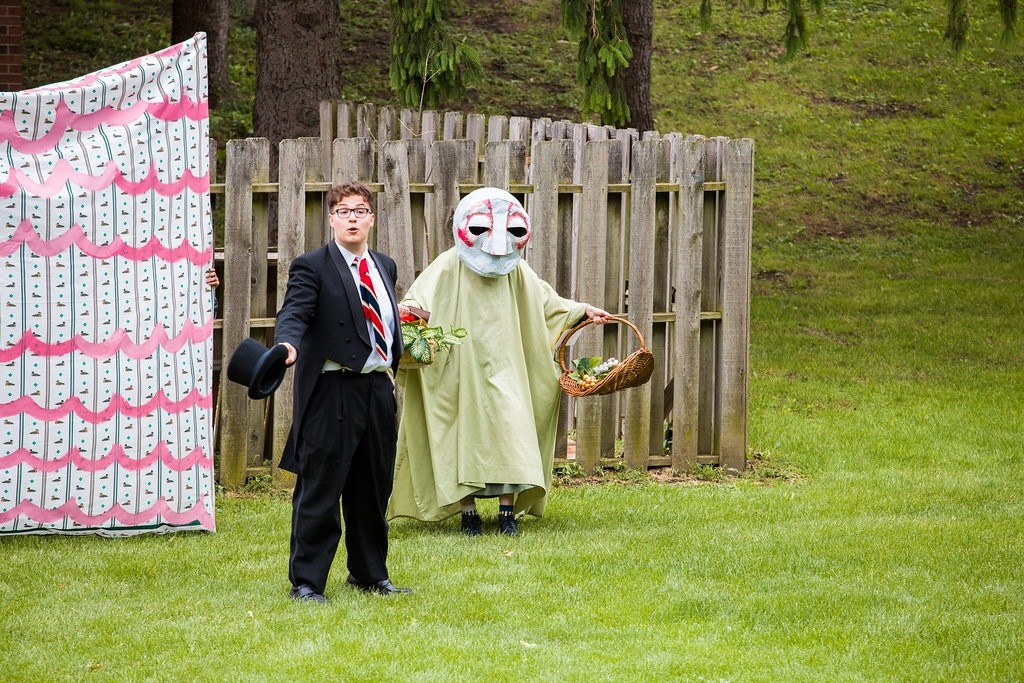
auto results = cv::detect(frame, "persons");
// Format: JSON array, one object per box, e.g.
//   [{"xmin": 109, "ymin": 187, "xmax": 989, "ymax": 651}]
[
  {"xmin": 403, "ymin": 187, "xmax": 612, "ymax": 533},
  {"xmin": 273, "ymin": 182, "xmax": 410, "ymax": 599}
]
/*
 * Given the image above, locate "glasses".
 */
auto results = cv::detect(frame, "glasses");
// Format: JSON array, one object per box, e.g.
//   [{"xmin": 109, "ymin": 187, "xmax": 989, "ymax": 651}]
[{"xmin": 330, "ymin": 208, "xmax": 371, "ymax": 218}]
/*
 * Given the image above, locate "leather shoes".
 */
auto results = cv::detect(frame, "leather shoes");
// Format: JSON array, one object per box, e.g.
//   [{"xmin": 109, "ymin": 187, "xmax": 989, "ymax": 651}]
[
  {"xmin": 288, "ymin": 585, "xmax": 333, "ymax": 605},
  {"xmin": 345, "ymin": 573, "xmax": 412, "ymax": 595}
]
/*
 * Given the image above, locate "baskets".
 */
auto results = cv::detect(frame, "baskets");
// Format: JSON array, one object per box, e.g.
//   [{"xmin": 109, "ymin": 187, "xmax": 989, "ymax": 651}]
[{"xmin": 559, "ymin": 316, "xmax": 655, "ymax": 396}]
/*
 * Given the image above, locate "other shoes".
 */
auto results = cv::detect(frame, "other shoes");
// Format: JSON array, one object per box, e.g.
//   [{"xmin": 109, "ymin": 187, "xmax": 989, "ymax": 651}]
[
  {"xmin": 498, "ymin": 514, "xmax": 518, "ymax": 536},
  {"xmin": 461, "ymin": 514, "xmax": 482, "ymax": 536}
]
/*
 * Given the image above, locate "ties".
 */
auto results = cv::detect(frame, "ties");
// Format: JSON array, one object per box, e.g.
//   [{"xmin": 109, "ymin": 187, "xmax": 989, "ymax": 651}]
[{"xmin": 351, "ymin": 257, "xmax": 388, "ymax": 362}]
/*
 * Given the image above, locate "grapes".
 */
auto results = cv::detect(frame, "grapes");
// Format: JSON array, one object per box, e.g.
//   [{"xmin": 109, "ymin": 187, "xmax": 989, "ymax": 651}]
[{"xmin": 579, "ymin": 375, "xmax": 598, "ymax": 390}]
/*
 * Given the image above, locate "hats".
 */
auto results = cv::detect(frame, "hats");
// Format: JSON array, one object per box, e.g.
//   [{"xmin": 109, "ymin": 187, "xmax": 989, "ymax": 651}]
[{"xmin": 226, "ymin": 337, "xmax": 289, "ymax": 400}]
